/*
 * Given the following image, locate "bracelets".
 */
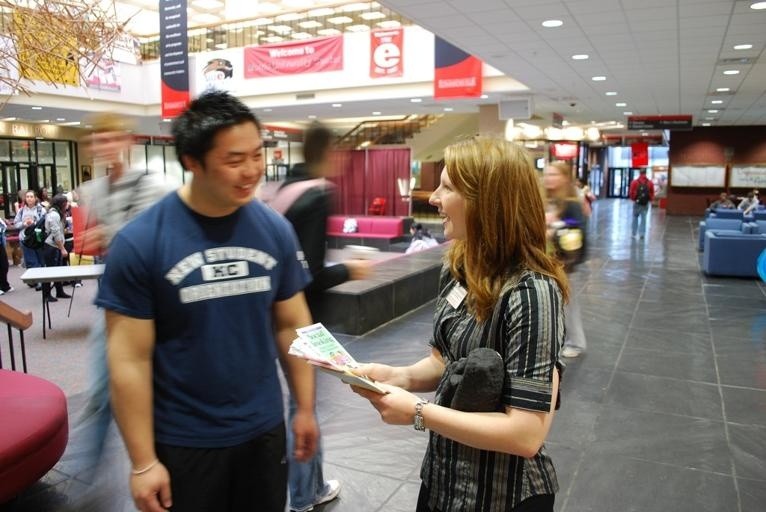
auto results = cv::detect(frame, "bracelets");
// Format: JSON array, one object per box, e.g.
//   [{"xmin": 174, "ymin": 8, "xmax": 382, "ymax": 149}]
[
  {"xmin": 131, "ymin": 460, "xmax": 161, "ymax": 475},
  {"xmin": 413, "ymin": 398, "xmax": 430, "ymax": 433}
]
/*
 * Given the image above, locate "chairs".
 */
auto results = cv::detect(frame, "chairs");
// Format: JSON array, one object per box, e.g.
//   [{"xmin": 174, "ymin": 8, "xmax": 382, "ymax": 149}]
[
  {"xmin": 696, "ymin": 196, "xmax": 766, "ymax": 281},
  {"xmin": 327, "ymin": 216, "xmax": 405, "ymax": 254}
]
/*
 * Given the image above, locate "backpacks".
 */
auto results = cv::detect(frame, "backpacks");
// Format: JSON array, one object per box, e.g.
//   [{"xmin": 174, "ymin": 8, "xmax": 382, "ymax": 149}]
[
  {"xmin": 21, "ymin": 205, "xmax": 64, "ymax": 249},
  {"xmin": 634, "ymin": 178, "xmax": 650, "ymax": 207}
]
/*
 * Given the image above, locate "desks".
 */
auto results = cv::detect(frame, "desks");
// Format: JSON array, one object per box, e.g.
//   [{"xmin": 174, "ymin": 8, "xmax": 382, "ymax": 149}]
[
  {"xmin": 411, "ymin": 191, "xmax": 439, "ymax": 218},
  {"xmin": 20, "ymin": 263, "xmax": 111, "ymax": 339}
]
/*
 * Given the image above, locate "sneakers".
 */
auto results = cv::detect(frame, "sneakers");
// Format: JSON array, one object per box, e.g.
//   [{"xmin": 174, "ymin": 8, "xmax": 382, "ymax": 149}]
[
  {"xmin": 46, "ymin": 292, "xmax": 58, "ymax": 302},
  {"xmin": 287, "ymin": 479, "xmax": 342, "ymax": 512},
  {"xmin": 28, "ymin": 282, "xmax": 42, "ymax": 292},
  {"xmin": 0, "ymin": 284, "xmax": 15, "ymax": 295},
  {"xmin": 74, "ymin": 282, "xmax": 84, "ymax": 288},
  {"xmin": 561, "ymin": 345, "xmax": 585, "ymax": 358}
]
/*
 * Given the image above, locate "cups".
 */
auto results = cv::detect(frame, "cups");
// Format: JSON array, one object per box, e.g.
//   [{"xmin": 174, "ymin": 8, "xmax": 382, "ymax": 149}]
[{"xmin": 397, "ymin": 176, "xmax": 416, "ymax": 201}]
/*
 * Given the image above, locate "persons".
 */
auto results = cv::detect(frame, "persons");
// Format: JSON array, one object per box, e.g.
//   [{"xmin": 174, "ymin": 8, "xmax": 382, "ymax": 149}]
[
  {"xmin": 349, "ymin": 136, "xmax": 567, "ymax": 512},
  {"xmin": 542, "ymin": 160, "xmax": 587, "ymax": 358},
  {"xmin": 629, "ymin": 168, "xmax": 654, "ymax": 239},
  {"xmin": 86, "ymin": 89, "xmax": 319, "ymax": 512},
  {"xmin": 575, "ymin": 178, "xmax": 596, "ymax": 226},
  {"xmin": 55, "ymin": 114, "xmax": 178, "ymax": 493},
  {"xmin": 405, "ymin": 221, "xmax": 438, "ymax": 255},
  {"xmin": 0, "ymin": 186, "xmax": 85, "ymax": 303},
  {"xmin": 709, "ymin": 189, "xmax": 762, "ymax": 215},
  {"xmin": 269, "ymin": 122, "xmax": 373, "ymax": 512}
]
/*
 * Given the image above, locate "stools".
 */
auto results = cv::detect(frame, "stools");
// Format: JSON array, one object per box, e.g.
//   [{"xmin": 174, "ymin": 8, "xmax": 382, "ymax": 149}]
[{"xmin": 0, "ymin": 367, "xmax": 70, "ymax": 505}]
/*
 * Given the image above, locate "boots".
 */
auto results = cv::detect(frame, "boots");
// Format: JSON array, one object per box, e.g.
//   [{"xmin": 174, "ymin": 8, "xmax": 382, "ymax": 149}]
[{"xmin": 54, "ymin": 281, "xmax": 72, "ymax": 299}]
